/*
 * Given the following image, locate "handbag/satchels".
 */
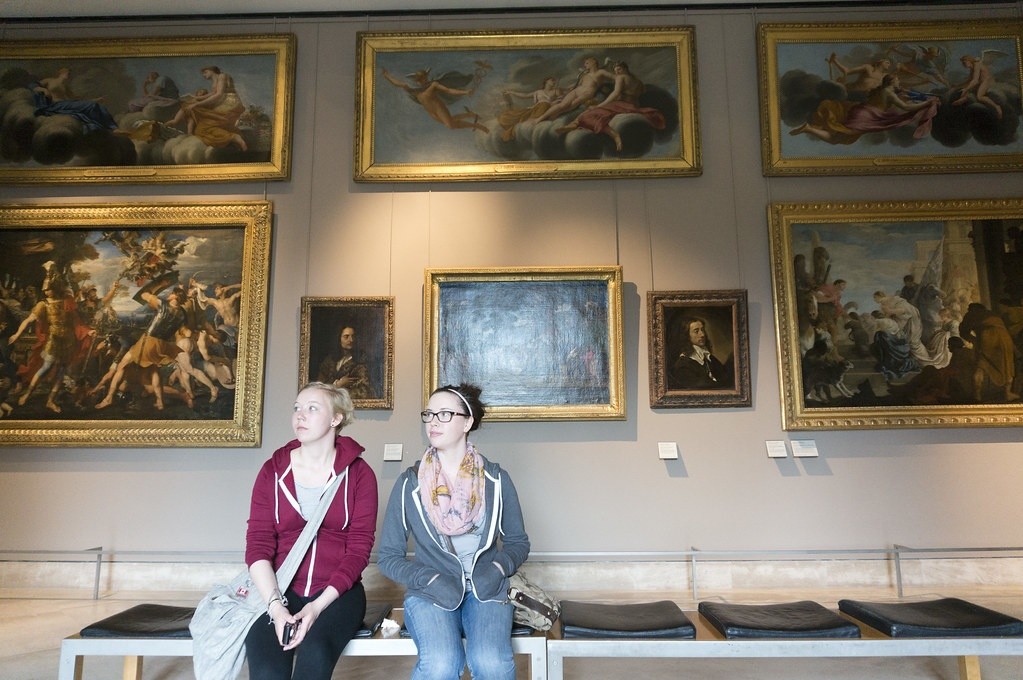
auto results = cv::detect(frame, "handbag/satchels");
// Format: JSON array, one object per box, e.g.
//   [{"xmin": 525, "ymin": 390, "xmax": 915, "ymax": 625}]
[
  {"xmin": 187, "ymin": 564, "xmax": 270, "ymax": 680},
  {"xmin": 505, "ymin": 570, "xmax": 561, "ymax": 631}
]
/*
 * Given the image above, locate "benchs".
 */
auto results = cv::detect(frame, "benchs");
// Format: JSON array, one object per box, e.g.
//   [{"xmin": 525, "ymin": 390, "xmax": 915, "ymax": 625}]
[
  {"xmin": 546, "ymin": 593, "xmax": 1023, "ymax": 680},
  {"xmin": 58, "ymin": 600, "xmax": 548, "ymax": 680}
]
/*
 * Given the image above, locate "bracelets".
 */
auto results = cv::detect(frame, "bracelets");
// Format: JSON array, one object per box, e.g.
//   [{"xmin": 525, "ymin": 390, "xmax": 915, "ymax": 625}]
[{"xmin": 266, "ymin": 591, "xmax": 288, "ymax": 625}]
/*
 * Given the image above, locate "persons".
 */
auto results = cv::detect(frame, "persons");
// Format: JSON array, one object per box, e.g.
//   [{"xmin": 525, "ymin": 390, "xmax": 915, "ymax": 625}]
[
  {"xmin": 245, "ymin": 381, "xmax": 379, "ymax": 680},
  {"xmin": 377, "ymin": 382, "xmax": 531, "ymax": 680}
]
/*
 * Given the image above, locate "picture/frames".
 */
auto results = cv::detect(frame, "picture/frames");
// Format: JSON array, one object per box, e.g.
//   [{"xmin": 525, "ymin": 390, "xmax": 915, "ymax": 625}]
[
  {"xmin": 422, "ymin": 264, "xmax": 628, "ymax": 422},
  {"xmin": 0, "ymin": 200, "xmax": 274, "ymax": 448},
  {"xmin": 299, "ymin": 295, "xmax": 394, "ymax": 410},
  {"xmin": 0, "ymin": 31, "xmax": 297, "ymax": 188},
  {"xmin": 647, "ymin": 288, "xmax": 752, "ymax": 410},
  {"xmin": 755, "ymin": 17, "xmax": 1023, "ymax": 177},
  {"xmin": 354, "ymin": 23, "xmax": 705, "ymax": 184},
  {"xmin": 766, "ymin": 196, "xmax": 1023, "ymax": 432}
]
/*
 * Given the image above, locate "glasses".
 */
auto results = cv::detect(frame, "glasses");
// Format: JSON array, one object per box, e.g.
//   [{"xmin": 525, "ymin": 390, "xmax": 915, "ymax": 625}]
[{"xmin": 421, "ymin": 411, "xmax": 471, "ymax": 423}]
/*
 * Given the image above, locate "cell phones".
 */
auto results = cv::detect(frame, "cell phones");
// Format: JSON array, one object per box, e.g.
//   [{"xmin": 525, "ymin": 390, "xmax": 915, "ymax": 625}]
[{"xmin": 283, "ymin": 619, "xmax": 301, "ymax": 644}]
